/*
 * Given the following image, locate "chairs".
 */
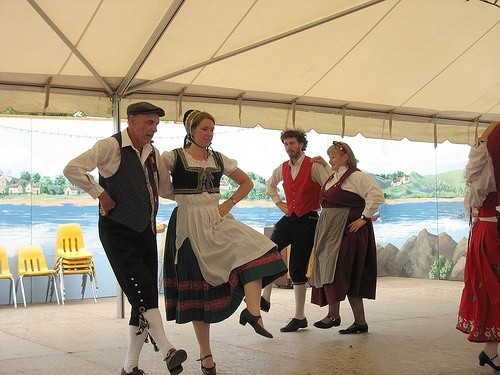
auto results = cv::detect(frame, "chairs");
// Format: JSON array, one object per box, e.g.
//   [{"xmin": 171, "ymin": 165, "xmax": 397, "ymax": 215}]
[{"xmin": 0, "ymin": 222, "xmax": 98, "ymax": 308}]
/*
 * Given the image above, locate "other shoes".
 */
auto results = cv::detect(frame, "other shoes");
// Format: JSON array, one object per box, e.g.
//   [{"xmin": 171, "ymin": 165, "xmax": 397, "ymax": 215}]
[
  {"xmin": 338, "ymin": 322, "xmax": 368, "ymax": 334},
  {"xmin": 120, "ymin": 366, "xmax": 147, "ymax": 375},
  {"xmin": 313, "ymin": 314, "xmax": 341, "ymax": 329},
  {"xmin": 163, "ymin": 348, "xmax": 187, "ymax": 375}
]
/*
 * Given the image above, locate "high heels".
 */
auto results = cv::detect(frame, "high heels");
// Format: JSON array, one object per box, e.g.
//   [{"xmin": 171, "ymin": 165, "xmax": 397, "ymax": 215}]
[
  {"xmin": 239, "ymin": 308, "xmax": 273, "ymax": 338},
  {"xmin": 478, "ymin": 350, "xmax": 500, "ymax": 372},
  {"xmin": 196, "ymin": 353, "xmax": 216, "ymax": 375}
]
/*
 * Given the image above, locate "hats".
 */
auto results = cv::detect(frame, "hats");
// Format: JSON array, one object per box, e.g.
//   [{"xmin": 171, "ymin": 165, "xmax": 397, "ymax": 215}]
[{"xmin": 127, "ymin": 102, "xmax": 165, "ymax": 118}]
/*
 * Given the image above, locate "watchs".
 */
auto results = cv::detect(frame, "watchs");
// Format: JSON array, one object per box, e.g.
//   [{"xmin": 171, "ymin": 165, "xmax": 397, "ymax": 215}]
[
  {"xmin": 229, "ymin": 197, "xmax": 236, "ymax": 205},
  {"xmin": 360, "ymin": 214, "xmax": 370, "ymax": 222}
]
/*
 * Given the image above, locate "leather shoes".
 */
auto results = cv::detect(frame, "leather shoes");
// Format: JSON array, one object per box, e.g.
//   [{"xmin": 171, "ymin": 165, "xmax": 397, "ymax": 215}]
[
  {"xmin": 280, "ymin": 318, "xmax": 308, "ymax": 332},
  {"xmin": 244, "ymin": 296, "xmax": 270, "ymax": 312}
]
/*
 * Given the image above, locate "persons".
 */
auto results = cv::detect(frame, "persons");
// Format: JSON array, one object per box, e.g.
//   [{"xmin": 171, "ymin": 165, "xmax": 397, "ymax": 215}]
[
  {"xmin": 162, "ymin": 109, "xmax": 288, "ymax": 375},
  {"xmin": 244, "ymin": 130, "xmax": 329, "ymax": 333},
  {"xmin": 310, "ymin": 140, "xmax": 385, "ymax": 335},
  {"xmin": 456, "ymin": 121, "xmax": 500, "ymax": 371},
  {"xmin": 63, "ymin": 102, "xmax": 187, "ymax": 375}
]
